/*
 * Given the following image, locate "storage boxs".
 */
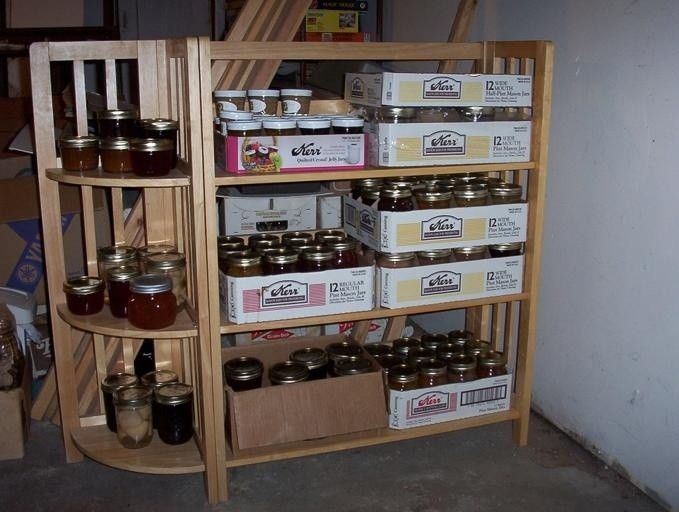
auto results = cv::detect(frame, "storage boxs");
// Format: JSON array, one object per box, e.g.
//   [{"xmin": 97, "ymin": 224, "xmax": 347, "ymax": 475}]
[{"xmin": 0, "ymin": 0, "xmax": 88, "ymax": 313}]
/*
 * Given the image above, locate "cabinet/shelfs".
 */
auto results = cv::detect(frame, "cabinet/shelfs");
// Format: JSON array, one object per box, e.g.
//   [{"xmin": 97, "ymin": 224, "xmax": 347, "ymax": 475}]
[{"xmin": 28, "ymin": 35, "xmax": 556, "ymax": 503}]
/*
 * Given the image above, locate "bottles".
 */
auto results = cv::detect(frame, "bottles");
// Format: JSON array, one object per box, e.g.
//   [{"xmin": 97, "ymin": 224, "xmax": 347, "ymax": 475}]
[
  {"xmin": 66, "ymin": 243, "xmax": 187, "ymax": 328},
  {"xmin": 352, "ymin": 175, "xmax": 521, "ymax": 268},
  {"xmin": 366, "ymin": 106, "xmax": 498, "ymax": 122},
  {"xmin": 228, "ymin": 330, "xmax": 507, "ymax": 391},
  {"xmin": 103, "ymin": 370, "xmax": 193, "ymax": 449},
  {"xmin": 213, "ymin": 89, "xmax": 364, "ymax": 136},
  {"xmin": 61, "ymin": 110, "xmax": 179, "ymax": 176},
  {"xmin": 220, "ymin": 232, "xmax": 356, "ymax": 278}
]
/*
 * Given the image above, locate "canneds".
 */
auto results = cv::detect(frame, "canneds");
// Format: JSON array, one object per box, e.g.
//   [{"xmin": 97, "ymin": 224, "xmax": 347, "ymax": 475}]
[
  {"xmin": 217, "ymin": 230, "xmax": 376, "ymax": 277},
  {"xmin": 351, "ymin": 103, "xmax": 531, "ymax": 124},
  {"xmin": 213, "ymin": 88, "xmax": 364, "ymax": 136},
  {"xmin": 101, "ymin": 370, "xmax": 194, "ymax": 449},
  {"xmin": 223, "ymin": 330, "xmax": 507, "ymax": 393},
  {"xmin": 61, "ymin": 108, "xmax": 180, "ymax": 178},
  {"xmin": 63, "ymin": 243, "xmax": 188, "ymax": 329},
  {"xmin": 348, "ymin": 233, "xmax": 525, "ymax": 269},
  {"xmin": 352, "ymin": 172, "xmax": 522, "ymax": 212}
]
